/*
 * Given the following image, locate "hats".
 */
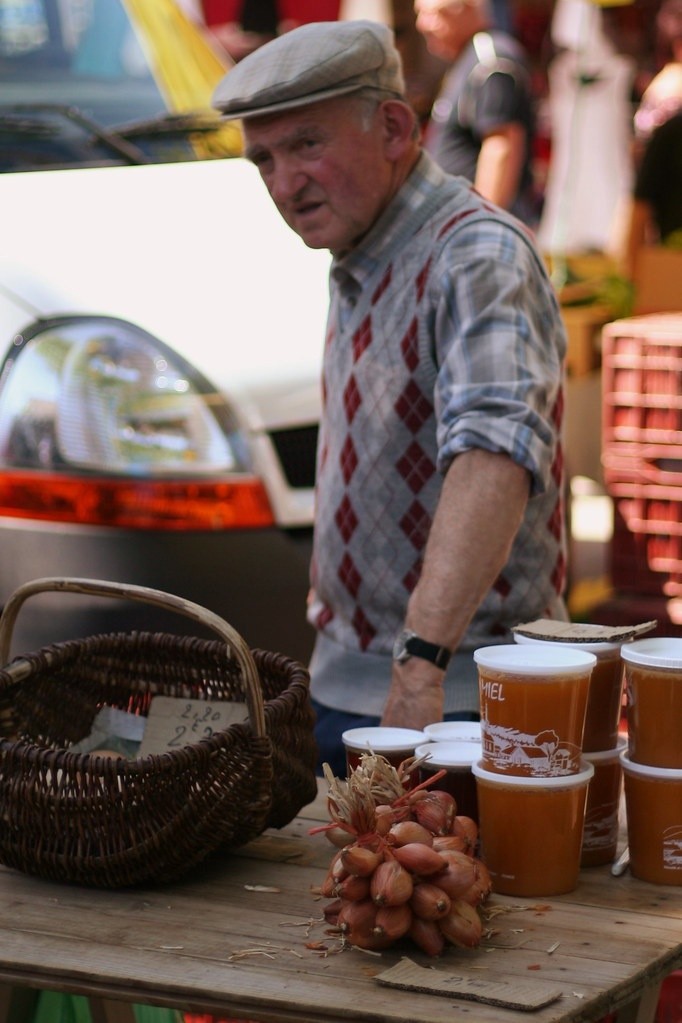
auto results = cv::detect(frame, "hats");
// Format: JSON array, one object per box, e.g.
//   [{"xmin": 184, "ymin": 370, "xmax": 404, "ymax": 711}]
[{"xmin": 211, "ymin": 20, "xmax": 400, "ymax": 121}]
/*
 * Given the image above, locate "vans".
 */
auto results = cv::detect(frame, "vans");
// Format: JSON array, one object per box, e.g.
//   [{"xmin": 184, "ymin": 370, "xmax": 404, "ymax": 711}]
[{"xmin": 2, "ymin": 0, "xmax": 333, "ymax": 673}]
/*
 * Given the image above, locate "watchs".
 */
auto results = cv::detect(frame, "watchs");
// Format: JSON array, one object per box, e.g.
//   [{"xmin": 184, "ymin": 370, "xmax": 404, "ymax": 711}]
[{"xmin": 391, "ymin": 628, "xmax": 453, "ymax": 671}]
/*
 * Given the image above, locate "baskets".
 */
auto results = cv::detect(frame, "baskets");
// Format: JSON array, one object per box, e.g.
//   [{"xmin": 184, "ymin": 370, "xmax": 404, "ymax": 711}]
[{"xmin": 0, "ymin": 578, "xmax": 311, "ymax": 884}]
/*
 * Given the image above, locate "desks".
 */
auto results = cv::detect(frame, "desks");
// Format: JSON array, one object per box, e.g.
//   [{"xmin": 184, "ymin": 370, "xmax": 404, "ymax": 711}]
[{"xmin": 0, "ymin": 773, "xmax": 682, "ymax": 1023}]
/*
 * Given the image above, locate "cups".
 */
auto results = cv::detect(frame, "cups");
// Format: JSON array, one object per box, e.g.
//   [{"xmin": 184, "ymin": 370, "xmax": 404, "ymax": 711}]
[{"xmin": 340, "ymin": 634, "xmax": 682, "ymax": 896}]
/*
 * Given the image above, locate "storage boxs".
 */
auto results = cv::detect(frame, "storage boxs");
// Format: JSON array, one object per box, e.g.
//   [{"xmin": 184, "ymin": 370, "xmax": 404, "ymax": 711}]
[{"xmin": 599, "ymin": 309, "xmax": 682, "ymax": 637}]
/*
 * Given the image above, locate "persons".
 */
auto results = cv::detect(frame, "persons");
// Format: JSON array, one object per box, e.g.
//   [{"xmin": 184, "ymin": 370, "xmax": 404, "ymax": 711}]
[
  {"xmin": 414, "ymin": 1, "xmax": 550, "ymax": 217},
  {"xmin": 533, "ymin": 0, "xmax": 682, "ymax": 266},
  {"xmin": 212, "ymin": 21, "xmax": 568, "ymax": 786}
]
